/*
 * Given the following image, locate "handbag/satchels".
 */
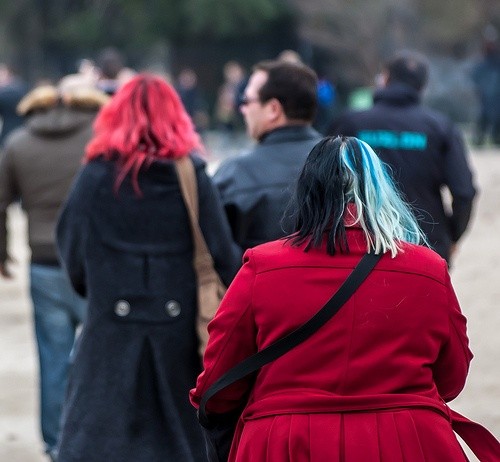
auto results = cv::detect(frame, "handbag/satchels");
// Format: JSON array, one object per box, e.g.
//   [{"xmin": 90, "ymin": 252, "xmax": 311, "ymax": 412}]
[
  {"xmin": 194, "ymin": 269, "xmax": 227, "ymax": 357},
  {"xmin": 197, "ymin": 388, "xmax": 252, "ymax": 462}
]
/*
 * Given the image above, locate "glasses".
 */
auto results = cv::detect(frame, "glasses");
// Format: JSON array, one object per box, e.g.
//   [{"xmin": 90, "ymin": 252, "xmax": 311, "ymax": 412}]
[{"xmin": 240, "ymin": 96, "xmax": 260, "ymax": 106}]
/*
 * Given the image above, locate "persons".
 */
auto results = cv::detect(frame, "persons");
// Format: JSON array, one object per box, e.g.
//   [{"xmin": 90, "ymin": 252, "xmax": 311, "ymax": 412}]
[
  {"xmin": 0, "ymin": 19, "xmax": 500, "ymax": 202},
  {"xmin": 210, "ymin": 59, "xmax": 332, "ymax": 254},
  {"xmin": 190, "ymin": 135, "xmax": 499, "ymax": 462},
  {"xmin": 324, "ymin": 47, "xmax": 478, "ymax": 272},
  {"xmin": 0, "ymin": 39, "xmax": 117, "ymax": 462},
  {"xmin": 55, "ymin": 75, "xmax": 247, "ymax": 462}
]
